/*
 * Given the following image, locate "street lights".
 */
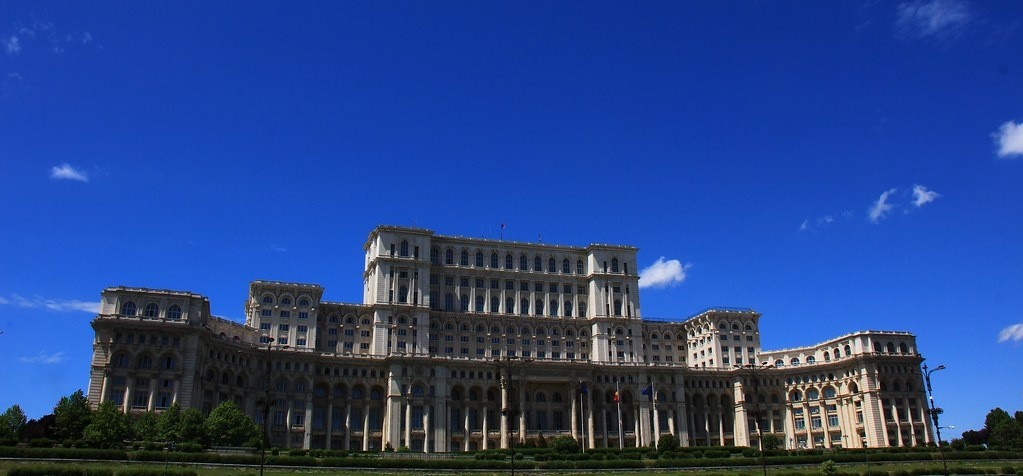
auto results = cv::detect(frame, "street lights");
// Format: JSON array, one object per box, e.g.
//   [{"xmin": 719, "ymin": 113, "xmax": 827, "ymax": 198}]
[
  {"xmin": 733, "ymin": 360, "xmax": 774, "ymax": 476},
  {"xmin": 494, "ymin": 354, "xmax": 535, "ymax": 476},
  {"xmin": 236, "ymin": 338, "xmax": 291, "ymax": 475},
  {"xmin": 910, "ymin": 363, "xmax": 955, "ymax": 476}
]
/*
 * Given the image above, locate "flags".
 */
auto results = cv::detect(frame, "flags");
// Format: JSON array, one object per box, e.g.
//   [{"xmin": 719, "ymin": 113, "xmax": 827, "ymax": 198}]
[
  {"xmin": 614, "ymin": 389, "xmax": 621, "ymax": 402},
  {"xmin": 580, "ymin": 384, "xmax": 588, "ymax": 394},
  {"xmin": 641, "ymin": 385, "xmax": 652, "ymax": 395}
]
